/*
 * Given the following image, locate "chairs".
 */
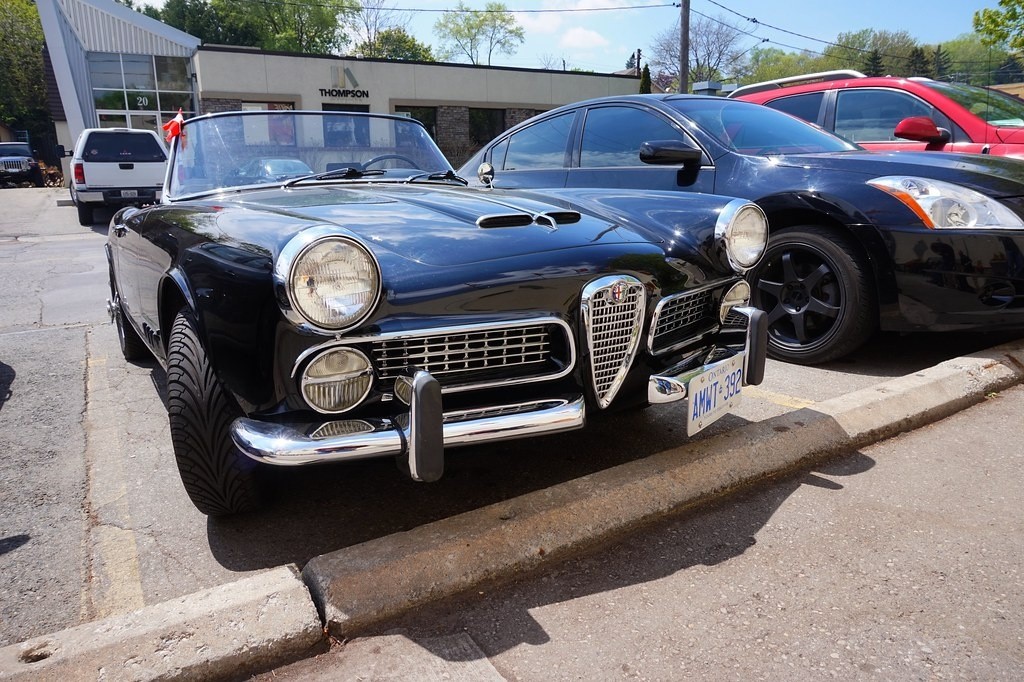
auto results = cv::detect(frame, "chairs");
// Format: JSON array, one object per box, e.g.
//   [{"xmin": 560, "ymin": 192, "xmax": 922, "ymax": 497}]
[{"xmin": 253, "ymin": 158, "xmax": 314, "ymax": 182}]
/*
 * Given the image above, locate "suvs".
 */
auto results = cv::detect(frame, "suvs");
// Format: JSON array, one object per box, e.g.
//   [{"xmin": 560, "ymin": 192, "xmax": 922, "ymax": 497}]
[
  {"xmin": 0, "ymin": 141, "xmax": 44, "ymax": 189},
  {"xmin": 716, "ymin": 67, "xmax": 1024, "ymax": 164},
  {"xmin": 55, "ymin": 126, "xmax": 187, "ymax": 225}
]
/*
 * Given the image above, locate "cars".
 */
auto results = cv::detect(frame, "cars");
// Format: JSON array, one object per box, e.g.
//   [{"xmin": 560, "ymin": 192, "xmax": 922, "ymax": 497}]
[
  {"xmin": 376, "ymin": 91, "xmax": 1024, "ymax": 366},
  {"xmin": 223, "ymin": 157, "xmax": 317, "ymax": 187},
  {"xmin": 104, "ymin": 109, "xmax": 772, "ymax": 521}
]
